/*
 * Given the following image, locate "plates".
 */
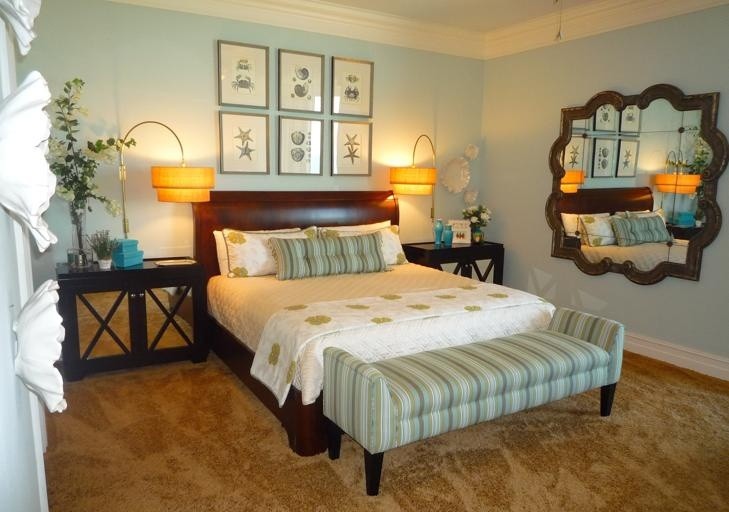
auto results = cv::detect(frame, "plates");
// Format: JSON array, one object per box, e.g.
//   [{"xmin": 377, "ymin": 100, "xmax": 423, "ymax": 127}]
[{"xmin": 155, "ymin": 259, "xmax": 198, "ymax": 266}]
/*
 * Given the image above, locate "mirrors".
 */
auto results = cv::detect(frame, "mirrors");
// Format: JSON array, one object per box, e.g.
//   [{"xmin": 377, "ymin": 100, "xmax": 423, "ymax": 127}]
[{"xmin": 545, "ymin": 82, "xmax": 726, "ymax": 286}]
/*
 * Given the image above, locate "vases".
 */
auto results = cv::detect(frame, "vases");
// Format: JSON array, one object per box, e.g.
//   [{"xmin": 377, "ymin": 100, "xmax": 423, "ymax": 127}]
[
  {"xmin": 69, "ymin": 201, "xmax": 93, "ymax": 268},
  {"xmin": 472, "ymin": 225, "xmax": 485, "ymax": 243}
]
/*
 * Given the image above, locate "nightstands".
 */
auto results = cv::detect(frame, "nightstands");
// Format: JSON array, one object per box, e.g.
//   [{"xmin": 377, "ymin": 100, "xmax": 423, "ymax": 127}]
[
  {"xmin": 670, "ymin": 222, "xmax": 702, "ymax": 240},
  {"xmin": 401, "ymin": 237, "xmax": 504, "ymax": 286},
  {"xmin": 55, "ymin": 256, "xmax": 207, "ymax": 382}
]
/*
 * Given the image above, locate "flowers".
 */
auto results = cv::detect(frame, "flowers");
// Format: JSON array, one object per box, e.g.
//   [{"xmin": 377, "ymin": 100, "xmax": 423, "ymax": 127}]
[
  {"xmin": 462, "ymin": 203, "xmax": 492, "ymax": 225},
  {"xmin": 666, "ymin": 126, "xmax": 711, "ymax": 200},
  {"xmin": 36, "ymin": 76, "xmax": 137, "ymax": 255}
]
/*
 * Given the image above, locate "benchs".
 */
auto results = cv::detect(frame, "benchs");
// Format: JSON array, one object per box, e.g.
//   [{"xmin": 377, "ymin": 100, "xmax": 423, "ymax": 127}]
[{"xmin": 319, "ymin": 306, "xmax": 628, "ymax": 497}]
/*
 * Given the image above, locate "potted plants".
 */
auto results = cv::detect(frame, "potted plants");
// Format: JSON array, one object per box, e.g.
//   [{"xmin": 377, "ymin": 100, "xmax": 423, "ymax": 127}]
[
  {"xmin": 86, "ymin": 231, "xmax": 117, "ymax": 270},
  {"xmin": 694, "ymin": 208, "xmax": 705, "ymax": 228}
]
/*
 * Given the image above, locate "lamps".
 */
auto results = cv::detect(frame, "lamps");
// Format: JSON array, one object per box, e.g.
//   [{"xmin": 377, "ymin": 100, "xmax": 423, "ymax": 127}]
[
  {"xmin": 653, "ymin": 149, "xmax": 700, "ymax": 209},
  {"xmin": 390, "ymin": 132, "xmax": 436, "ymax": 222},
  {"xmin": 119, "ymin": 120, "xmax": 217, "ymax": 237},
  {"xmin": 562, "ymin": 169, "xmax": 584, "ymax": 194}
]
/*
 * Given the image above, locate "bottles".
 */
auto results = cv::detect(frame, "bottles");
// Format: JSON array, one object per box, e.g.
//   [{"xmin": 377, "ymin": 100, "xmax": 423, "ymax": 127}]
[
  {"xmin": 433, "ymin": 219, "xmax": 444, "ymax": 245},
  {"xmin": 472, "ymin": 226, "xmax": 483, "ymax": 243},
  {"xmin": 442, "ymin": 224, "xmax": 453, "ymax": 245}
]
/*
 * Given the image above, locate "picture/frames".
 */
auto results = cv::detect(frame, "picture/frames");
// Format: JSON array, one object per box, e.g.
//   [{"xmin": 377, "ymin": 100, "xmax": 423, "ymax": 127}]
[
  {"xmin": 217, "ymin": 39, "xmax": 375, "ymax": 176},
  {"xmin": 565, "ymin": 103, "xmax": 643, "ymax": 178}
]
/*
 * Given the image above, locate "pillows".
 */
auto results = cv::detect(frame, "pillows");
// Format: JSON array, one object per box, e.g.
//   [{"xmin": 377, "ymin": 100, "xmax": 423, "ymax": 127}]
[
  {"xmin": 212, "ymin": 220, "xmax": 410, "ymax": 281},
  {"xmin": 559, "ymin": 209, "xmax": 672, "ymax": 245}
]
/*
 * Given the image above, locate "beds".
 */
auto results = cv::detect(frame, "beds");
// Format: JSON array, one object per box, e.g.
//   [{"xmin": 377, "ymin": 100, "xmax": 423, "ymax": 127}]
[
  {"xmin": 192, "ymin": 190, "xmax": 554, "ymax": 456},
  {"xmin": 552, "ymin": 186, "xmax": 691, "ymax": 265}
]
